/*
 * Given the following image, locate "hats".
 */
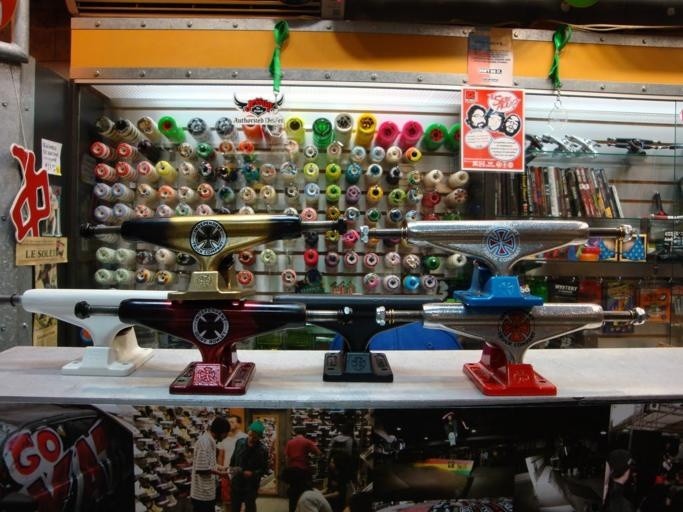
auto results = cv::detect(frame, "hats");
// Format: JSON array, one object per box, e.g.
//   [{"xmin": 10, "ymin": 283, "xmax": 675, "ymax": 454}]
[{"xmin": 247, "ymin": 421, "xmax": 266, "ymax": 439}]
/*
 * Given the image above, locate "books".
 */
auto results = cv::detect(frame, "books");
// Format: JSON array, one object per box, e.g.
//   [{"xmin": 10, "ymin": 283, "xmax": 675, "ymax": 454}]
[{"xmin": 494, "ymin": 166, "xmax": 624, "ymax": 219}]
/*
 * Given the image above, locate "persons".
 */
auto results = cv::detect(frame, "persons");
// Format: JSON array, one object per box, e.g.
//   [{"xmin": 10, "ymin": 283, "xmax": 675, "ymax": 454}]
[
  {"xmin": 216, "ymin": 416, "xmax": 248, "ymax": 512},
  {"xmin": 502, "ymin": 113, "xmax": 521, "ymax": 136},
  {"xmin": 598, "ymin": 440, "xmax": 683, "ymax": 512},
  {"xmin": 441, "ymin": 410, "xmax": 472, "ymax": 446},
  {"xmin": 190, "ymin": 417, "xmax": 231, "ymax": 512},
  {"xmin": 466, "ymin": 105, "xmax": 486, "ymax": 129},
  {"xmin": 230, "ymin": 420, "xmax": 269, "ymax": 512},
  {"xmin": 485, "ymin": 108, "xmax": 505, "ymax": 132},
  {"xmin": 284, "ymin": 409, "xmax": 396, "ymax": 510}
]
